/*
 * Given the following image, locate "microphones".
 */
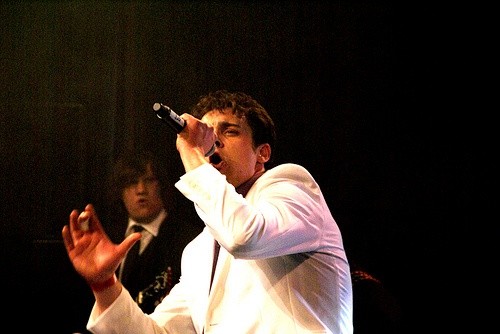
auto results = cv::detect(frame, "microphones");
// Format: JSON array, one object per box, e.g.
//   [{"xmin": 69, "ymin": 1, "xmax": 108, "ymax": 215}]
[{"xmin": 153, "ymin": 101, "xmax": 216, "ymax": 158}]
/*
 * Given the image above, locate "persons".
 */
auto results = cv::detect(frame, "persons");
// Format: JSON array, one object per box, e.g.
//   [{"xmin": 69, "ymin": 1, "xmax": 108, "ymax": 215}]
[
  {"xmin": 99, "ymin": 149, "xmax": 199, "ymax": 316},
  {"xmin": 62, "ymin": 90, "xmax": 354, "ymax": 334}
]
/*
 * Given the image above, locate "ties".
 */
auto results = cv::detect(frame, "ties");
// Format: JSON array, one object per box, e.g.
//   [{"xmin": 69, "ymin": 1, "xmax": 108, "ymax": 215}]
[{"xmin": 121, "ymin": 225, "xmax": 144, "ymax": 301}]
